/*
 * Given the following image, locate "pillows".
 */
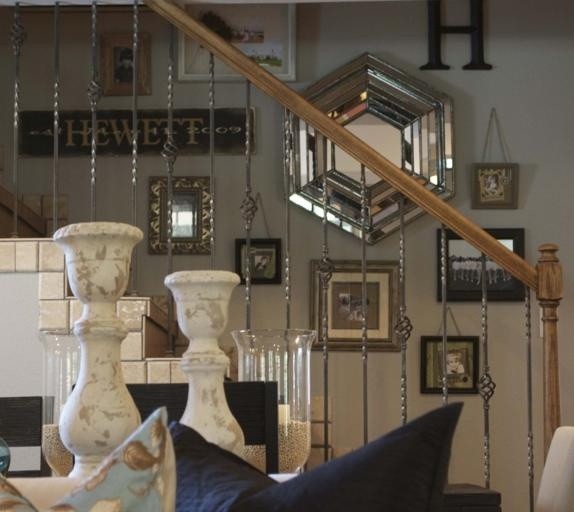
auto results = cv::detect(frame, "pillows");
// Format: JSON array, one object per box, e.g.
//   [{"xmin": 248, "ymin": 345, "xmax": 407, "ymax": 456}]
[
  {"xmin": 1, "ymin": 405, "xmax": 178, "ymax": 512},
  {"xmin": 169, "ymin": 401, "xmax": 465, "ymax": 511}
]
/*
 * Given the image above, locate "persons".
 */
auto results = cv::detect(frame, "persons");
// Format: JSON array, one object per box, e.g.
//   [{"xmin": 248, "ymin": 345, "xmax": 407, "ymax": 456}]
[
  {"xmin": 444, "ymin": 350, "xmax": 464, "ymax": 375},
  {"xmin": 255, "ymin": 257, "xmax": 268, "ymax": 271},
  {"xmin": 450, "ymin": 256, "xmax": 513, "ymax": 286}
]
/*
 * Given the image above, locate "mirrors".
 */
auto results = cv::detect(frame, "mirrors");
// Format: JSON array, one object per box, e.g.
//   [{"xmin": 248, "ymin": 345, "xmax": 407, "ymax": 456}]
[
  {"xmin": 148, "ymin": 176, "xmax": 211, "ymax": 255},
  {"xmin": 283, "ymin": 52, "xmax": 455, "ymax": 247}
]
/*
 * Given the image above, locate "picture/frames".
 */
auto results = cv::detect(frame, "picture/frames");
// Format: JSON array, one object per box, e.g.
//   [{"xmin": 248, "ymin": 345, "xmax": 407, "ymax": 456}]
[
  {"xmin": 235, "ymin": 238, "xmax": 281, "ymax": 284},
  {"xmin": 438, "ymin": 228, "xmax": 525, "ymax": 301},
  {"xmin": 178, "ymin": 0, "xmax": 296, "ymax": 82},
  {"xmin": 421, "ymin": 336, "xmax": 478, "ymax": 393},
  {"xmin": 471, "ymin": 162, "xmax": 518, "ymax": 209},
  {"xmin": 311, "ymin": 260, "xmax": 401, "ymax": 352},
  {"xmin": 100, "ymin": 32, "xmax": 151, "ymax": 96}
]
